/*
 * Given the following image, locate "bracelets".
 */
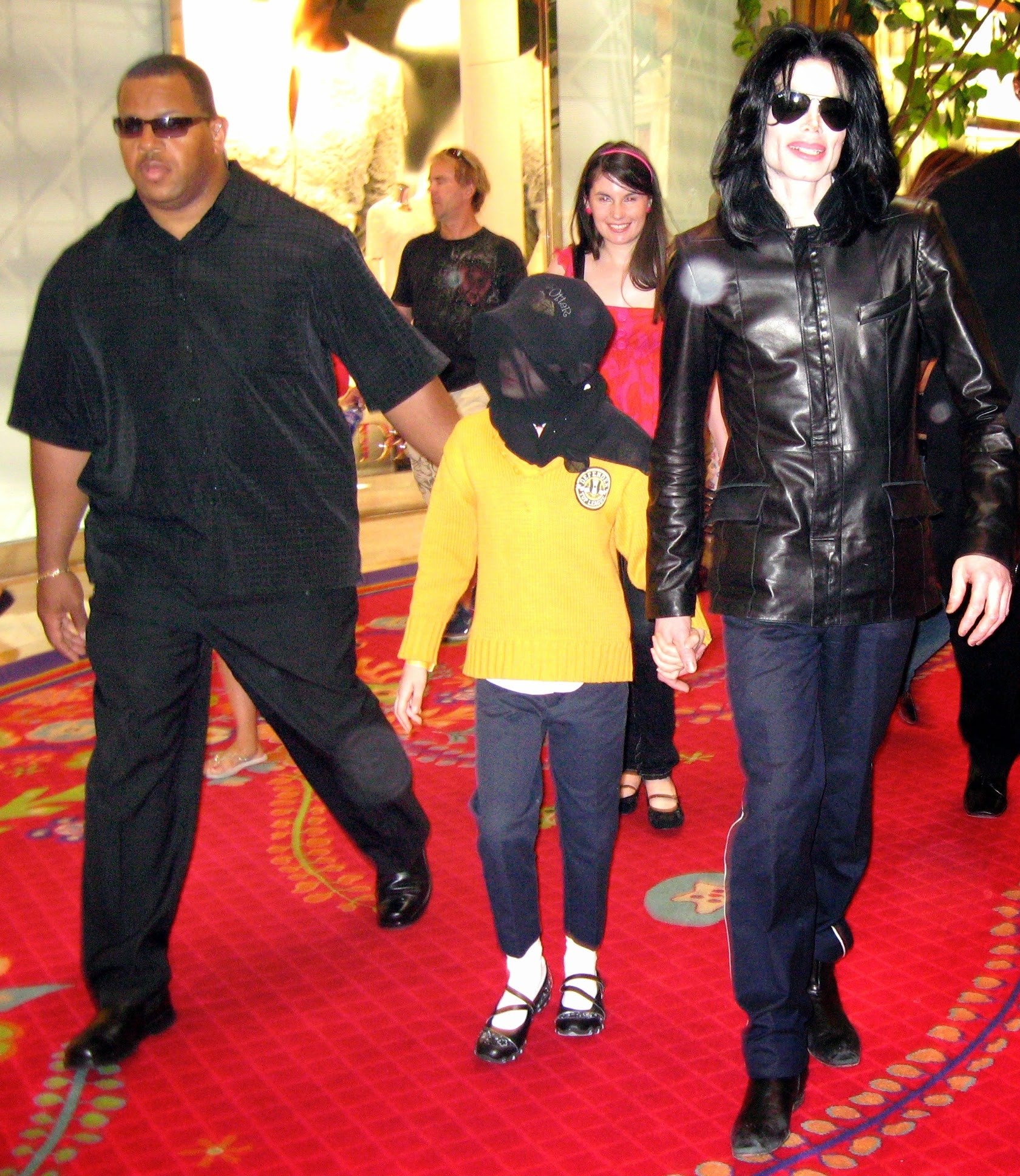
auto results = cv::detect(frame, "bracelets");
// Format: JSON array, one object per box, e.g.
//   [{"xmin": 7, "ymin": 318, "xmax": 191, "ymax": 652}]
[
  {"xmin": 36, "ymin": 564, "xmax": 71, "ymax": 583},
  {"xmin": 404, "ymin": 659, "xmax": 435, "ymax": 672}
]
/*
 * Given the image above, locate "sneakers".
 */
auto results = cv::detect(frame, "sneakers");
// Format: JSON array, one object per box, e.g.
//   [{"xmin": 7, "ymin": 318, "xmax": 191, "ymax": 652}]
[{"xmin": 442, "ymin": 603, "xmax": 474, "ymax": 641}]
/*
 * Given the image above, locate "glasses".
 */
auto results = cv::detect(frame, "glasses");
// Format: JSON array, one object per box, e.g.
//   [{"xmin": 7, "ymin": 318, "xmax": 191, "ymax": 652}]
[
  {"xmin": 111, "ymin": 115, "xmax": 205, "ymax": 139},
  {"xmin": 769, "ymin": 90, "xmax": 854, "ymax": 131},
  {"xmin": 440, "ymin": 147, "xmax": 472, "ymax": 168}
]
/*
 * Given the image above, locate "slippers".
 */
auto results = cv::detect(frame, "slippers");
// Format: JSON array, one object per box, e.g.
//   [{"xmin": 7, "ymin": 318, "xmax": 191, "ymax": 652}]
[{"xmin": 206, "ymin": 750, "xmax": 269, "ymax": 779}]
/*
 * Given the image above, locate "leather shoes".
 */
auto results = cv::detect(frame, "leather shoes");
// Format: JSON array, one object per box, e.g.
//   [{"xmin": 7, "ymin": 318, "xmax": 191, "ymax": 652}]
[
  {"xmin": 62, "ymin": 982, "xmax": 176, "ymax": 1068},
  {"xmin": 618, "ymin": 773, "xmax": 642, "ymax": 814},
  {"xmin": 473, "ymin": 964, "xmax": 553, "ymax": 1062},
  {"xmin": 804, "ymin": 959, "xmax": 862, "ymax": 1068},
  {"xmin": 376, "ymin": 848, "xmax": 433, "ymax": 932},
  {"xmin": 554, "ymin": 968, "xmax": 606, "ymax": 1037},
  {"xmin": 644, "ymin": 786, "xmax": 685, "ymax": 830},
  {"xmin": 962, "ymin": 773, "xmax": 1010, "ymax": 818},
  {"xmin": 731, "ymin": 1065, "xmax": 806, "ymax": 1158}
]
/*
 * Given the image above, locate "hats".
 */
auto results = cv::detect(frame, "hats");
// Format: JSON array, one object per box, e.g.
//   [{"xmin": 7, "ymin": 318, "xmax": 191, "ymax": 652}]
[{"xmin": 486, "ymin": 274, "xmax": 616, "ymax": 371}]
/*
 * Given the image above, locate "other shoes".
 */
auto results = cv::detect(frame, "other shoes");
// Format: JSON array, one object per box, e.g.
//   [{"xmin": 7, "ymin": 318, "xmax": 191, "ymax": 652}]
[{"xmin": 899, "ymin": 694, "xmax": 918, "ymax": 726}]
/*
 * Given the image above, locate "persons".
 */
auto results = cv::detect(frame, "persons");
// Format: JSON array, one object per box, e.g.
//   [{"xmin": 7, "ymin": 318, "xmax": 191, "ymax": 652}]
[
  {"xmin": 921, "ymin": 60, "xmax": 1019, "ymax": 821},
  {"xmin": 389, "ymin": 144, "xmax": 533, "ymax": 644},
  {"xmin": 640, "ymin": 19, "xmax": 1020, "ymax": 1160},
  {"xmin": 542, "ymin": 136, "xmax": 732, "ymax": 833},
  {"xmin": 5, "ymin": 47, "xmax": 467, "ymax": 1075},
  {"xmin": 897, "ymin": 144, "xmax": 988, "ymax": 723},
  {"xmin": 391, "ymin": 271, "xmax": 715, "ymax": 1066},
  {"xmin": 205, "ymin": 646, "xmax": 271, "ymax": 782},
  {"xmin": 195, "ymin": 0, "xmax": 413, "ymax": 282}
]
